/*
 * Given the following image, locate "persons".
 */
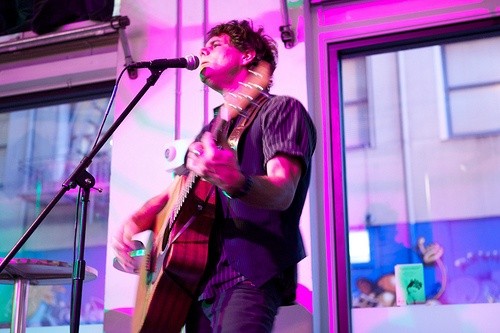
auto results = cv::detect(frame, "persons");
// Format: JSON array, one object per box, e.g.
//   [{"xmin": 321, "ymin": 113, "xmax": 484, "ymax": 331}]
[{"xmin": 112, "ymin": 19, "xmax": 317, "ymax": 333}]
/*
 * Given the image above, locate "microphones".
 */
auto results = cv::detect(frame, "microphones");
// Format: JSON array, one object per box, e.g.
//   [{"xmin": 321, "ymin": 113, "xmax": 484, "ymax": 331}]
[{"xmin": 129, "ymin": 55, "xmax": 199, "ymax": 70}]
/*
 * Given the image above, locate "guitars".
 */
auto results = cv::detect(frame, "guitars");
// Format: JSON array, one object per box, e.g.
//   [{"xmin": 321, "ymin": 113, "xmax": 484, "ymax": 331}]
[{"xmin": 129, "ymin": 61, "xmax": 271, "ymax": 333}]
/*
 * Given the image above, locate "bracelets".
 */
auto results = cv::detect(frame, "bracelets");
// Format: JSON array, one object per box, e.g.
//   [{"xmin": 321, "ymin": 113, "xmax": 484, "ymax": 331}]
[{"xmin": 222, "ymin": 172, "xmax": 255, "ymax": 199}]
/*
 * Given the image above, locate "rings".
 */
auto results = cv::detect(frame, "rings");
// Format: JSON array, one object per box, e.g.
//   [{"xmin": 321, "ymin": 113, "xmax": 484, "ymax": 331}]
[{"xmin": 193, "ymin": 146, "xmax": 203, "ymax": 153}]
